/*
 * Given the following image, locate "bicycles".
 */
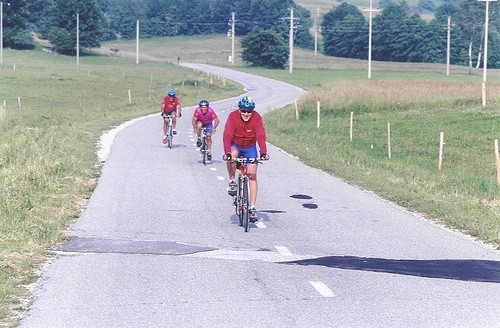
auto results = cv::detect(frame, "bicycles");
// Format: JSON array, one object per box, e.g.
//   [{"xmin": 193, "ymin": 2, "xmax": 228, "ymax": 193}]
[
  {"xmin": 198, "ymin": 129, "xmax": 213, "ymax": 164},
  {"xmin": 226, "ymin": 157, "xmax": 264, "ymax": 232},
  {"xmin": 160, "ymin": 112, "xmax": 182, "ymax": 149}
]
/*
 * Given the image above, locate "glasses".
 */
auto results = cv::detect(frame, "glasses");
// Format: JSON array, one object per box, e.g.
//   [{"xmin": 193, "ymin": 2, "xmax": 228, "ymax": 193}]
[
  {"xmin": 240, "ymin": 109, "xmax": 252, "ymax": 113},
  {"xmin": 170, "ymin": 96, "xmax": 174, "ymax": 97}
]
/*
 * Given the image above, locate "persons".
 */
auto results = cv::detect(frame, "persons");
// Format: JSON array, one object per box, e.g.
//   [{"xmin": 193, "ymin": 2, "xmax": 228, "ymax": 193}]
[
  {"xmin": 191, "ymin": 100, "xmax": 220, "ymax": 161},
  {"xmin": 161, "ymin": 89, "xmax": 182, "ymax": 144},
  {"xmin": 223, "ymin": 96, "xmax": 269, "ymax": 222}
]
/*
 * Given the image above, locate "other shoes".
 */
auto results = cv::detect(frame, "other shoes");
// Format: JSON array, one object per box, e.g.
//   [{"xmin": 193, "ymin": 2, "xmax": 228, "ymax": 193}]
[
  {"xmin": 196, "ymin": 139, "xmax": 202, "ymax": 147},
  {"xmin": 249, "ymin": 207, "xmax": 258, "ymax": 221},
  {"xmin": 207, "ymin": 153, "xmax": 211, "ymax": 160},
  {"xmin": 228, "ymin": 181, "xmax": 237, "ymax": 196},
  {"xmin": 173, "ymin": 131, "xmax": 176, "ymax": 134},
  {"xmin": 163, "ymin": 136, "xmax": 168, "ymax": 144}
]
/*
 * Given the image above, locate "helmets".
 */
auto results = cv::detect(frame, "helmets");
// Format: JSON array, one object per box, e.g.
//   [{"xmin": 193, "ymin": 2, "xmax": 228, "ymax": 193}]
[
  {"xmin": 199, "ymin": 100, "xmax": 209, "ymax": 106},
  {"xmin": 238, "ymin": 96, "xmax": 255, "ymax": 108},
  {"xmin": 168, "ymin": 89, "xmax": 176, "ymax": 96}
]
